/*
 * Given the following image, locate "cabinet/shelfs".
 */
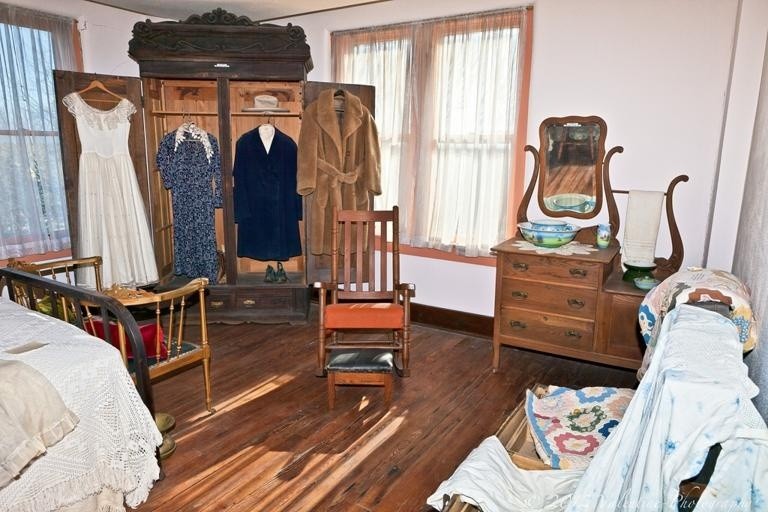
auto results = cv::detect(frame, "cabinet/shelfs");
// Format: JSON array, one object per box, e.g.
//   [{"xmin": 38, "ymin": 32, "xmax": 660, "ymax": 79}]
[
  {"xmin": 490, "ymin": 236, "xmax": 657, "ymax": 374},
  {"xmin": 52, "ymin": 69, "xmax": 375, "ymax": 326}
]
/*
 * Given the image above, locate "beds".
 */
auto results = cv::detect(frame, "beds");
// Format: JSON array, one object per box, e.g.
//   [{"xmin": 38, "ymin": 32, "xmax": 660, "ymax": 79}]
[{"xmin": 0, "ymin": 266, "xmax": 163, "ymax": 511}]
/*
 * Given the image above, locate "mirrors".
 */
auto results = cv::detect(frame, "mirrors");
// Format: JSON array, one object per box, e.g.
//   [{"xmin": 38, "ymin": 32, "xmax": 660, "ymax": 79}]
[{"xmin": 516, "ymin": 116, "xmax": 624, "ymax": 245}]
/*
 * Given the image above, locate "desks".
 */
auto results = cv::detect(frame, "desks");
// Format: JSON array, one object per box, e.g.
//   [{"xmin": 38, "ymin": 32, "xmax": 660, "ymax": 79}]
[{"xmin": 105, "ymin": 279, "xmax": 209, "ymax": 339}]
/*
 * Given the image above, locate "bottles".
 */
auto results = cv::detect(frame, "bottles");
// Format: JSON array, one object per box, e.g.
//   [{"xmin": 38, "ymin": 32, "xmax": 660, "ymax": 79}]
[{"xmin": 596, "ymin": 223, "xmax": 613, "ymax": 249}]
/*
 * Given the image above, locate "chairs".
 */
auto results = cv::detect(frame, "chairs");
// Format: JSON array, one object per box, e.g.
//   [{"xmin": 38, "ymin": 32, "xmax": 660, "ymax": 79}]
[
  {"xmin": 314, "ymin": 206, "xmax": 415, "ymax": 378},
  {"xmin": 6, "ymin": 255, "xmax": 217, "ymax": 415}
]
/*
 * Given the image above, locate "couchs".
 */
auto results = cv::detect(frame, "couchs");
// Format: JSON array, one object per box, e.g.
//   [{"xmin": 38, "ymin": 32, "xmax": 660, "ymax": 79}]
[{"xmin": 443, "ymin": 266, "xmax": 768, "ymax": 511}]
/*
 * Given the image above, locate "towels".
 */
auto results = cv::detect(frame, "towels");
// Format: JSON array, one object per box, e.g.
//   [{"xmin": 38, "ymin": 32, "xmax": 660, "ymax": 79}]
[{"xmin": 623, "ymin": 190, "xmax": 664, "ymax": 267}]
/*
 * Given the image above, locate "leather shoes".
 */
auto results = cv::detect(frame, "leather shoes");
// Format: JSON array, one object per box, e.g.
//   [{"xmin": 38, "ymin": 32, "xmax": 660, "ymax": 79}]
[
  {"xmin": 155, "ymin": 412, "xmax": 176, "ymax": 433},
  {"xmin": 263, "ymin": 264, "xmax": 275, "ymax": 282},
  {"xmin": 276, "ymin": 261, "xmax": 287, "ymax": 283},
  {"xmin": 158, "ymin": 435, "xmax": 176, "ymax": 457}
]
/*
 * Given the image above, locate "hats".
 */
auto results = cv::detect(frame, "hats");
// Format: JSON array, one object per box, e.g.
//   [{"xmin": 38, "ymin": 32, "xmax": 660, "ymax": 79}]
[{"xmin": 241, "ymin": 94, "xmax": 290, "ymax": 112}]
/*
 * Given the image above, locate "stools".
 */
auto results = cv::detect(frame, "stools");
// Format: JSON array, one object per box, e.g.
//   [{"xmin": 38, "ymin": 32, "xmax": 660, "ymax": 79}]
[{"xmin": 324, "ymin": 348, "xmax": 393, "ymax": 411}]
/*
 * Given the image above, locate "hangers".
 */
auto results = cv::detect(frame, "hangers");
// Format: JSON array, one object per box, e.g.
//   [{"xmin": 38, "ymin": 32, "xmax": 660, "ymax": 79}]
[
  {"xmin": 171, "ymin": 110, "xmax": 202, "ymax": 142},
  {"xmin": 334, "ymin": 84, "xmax": 345, "ymax": 98},
  {"xmin": 258, "ymin": 111, "xmax": 273, "ymax": 131},
  {"xmin": 63, "ymin": 72, "xmax": 133, "ymax": 108}
]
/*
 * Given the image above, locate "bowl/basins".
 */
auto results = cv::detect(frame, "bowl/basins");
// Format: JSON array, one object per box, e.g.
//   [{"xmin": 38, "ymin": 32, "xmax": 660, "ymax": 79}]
[
  {"xmin": 633, "ymin": 277, "xmax": 662, "ymax": 290},
  {"xmin": 543, "ymin": 193, "xmax": 597, "ymax": 214},
  {"xmin": 518, "ymin": 220, "xmax": 582, "ymax": 248}
]
